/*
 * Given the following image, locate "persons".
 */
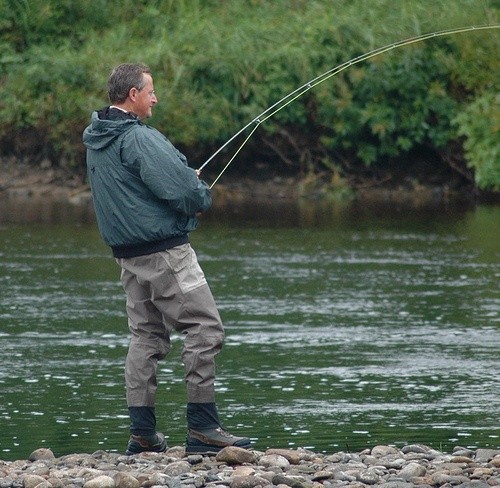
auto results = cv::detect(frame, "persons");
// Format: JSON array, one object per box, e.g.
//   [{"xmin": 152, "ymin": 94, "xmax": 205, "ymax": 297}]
[{"xmin": 82, "ymin": 60, "xmax": 255, "ymax": 455}]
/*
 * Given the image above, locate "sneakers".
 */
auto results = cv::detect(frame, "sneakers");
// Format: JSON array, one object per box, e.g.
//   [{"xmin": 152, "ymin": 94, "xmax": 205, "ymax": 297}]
[
  {"xmin": 125, "ymin": 432, "xmax": 168, "ymax": 454},
  {"xmin": 184, "ymin": 429, "xmax": 252, "ymax": 455}
]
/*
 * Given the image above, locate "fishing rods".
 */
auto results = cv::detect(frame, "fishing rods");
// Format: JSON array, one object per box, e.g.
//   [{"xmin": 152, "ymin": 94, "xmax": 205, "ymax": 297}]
[{"xmin": 195, "ymin": 24, "xmax": 499, "ymax": 190}]
